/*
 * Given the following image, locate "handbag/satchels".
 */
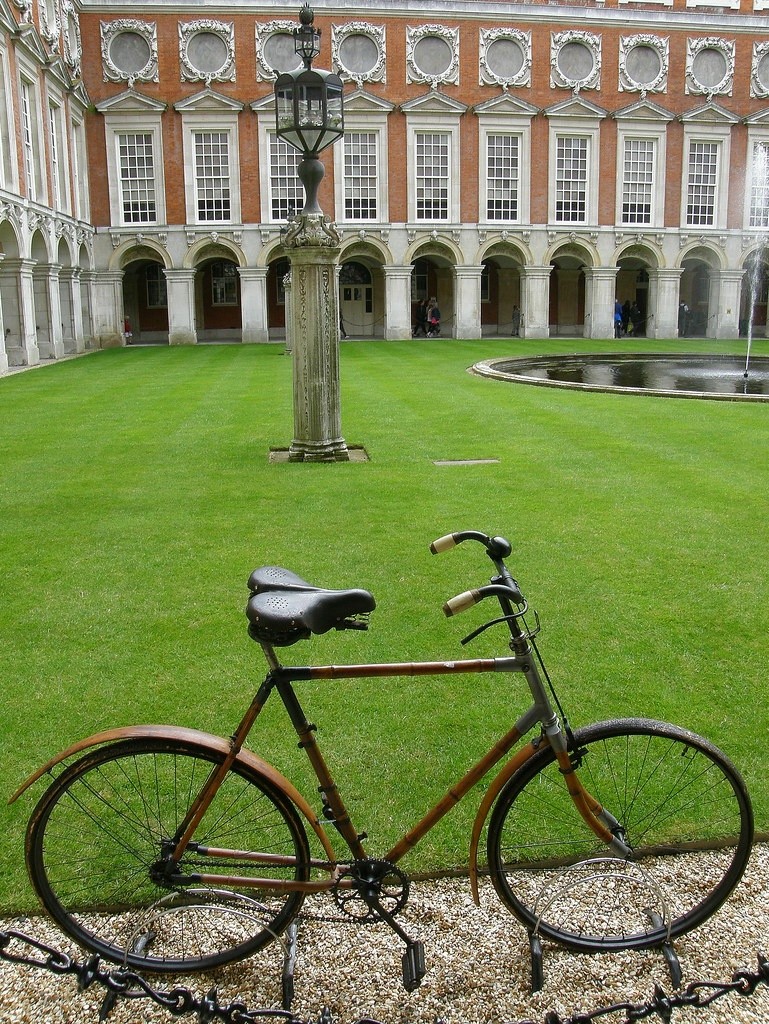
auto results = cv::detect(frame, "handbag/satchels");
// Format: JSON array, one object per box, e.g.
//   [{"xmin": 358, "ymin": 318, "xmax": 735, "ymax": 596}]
[
  {"xmin": 627, "ymin": 320, "xmax": 634, "ymax": 332},
  {"xmin": 432, "ymin": 317, "xmax": 437, "ymax": 324}
]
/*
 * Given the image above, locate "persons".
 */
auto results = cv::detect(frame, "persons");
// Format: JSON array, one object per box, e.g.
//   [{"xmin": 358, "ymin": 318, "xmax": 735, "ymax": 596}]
[
  {"xmin": 615, "ymin": 297, "xmax": 640, "ymax": 339},
  {"xmin": 124, "ymin": 315, "xmax": 135, "ymax": 345},
  {"xmin": 679, "ymin": 300, "xmax": 693, "ymax": 337},
  {"xmin": 511, "ymin": 305, "xmax": 520, "ymax": 336},
  {"xmin": 413, "ymin": 296, "xmax": 442, "ymax": 337},
  {"xmin": 339, "ymin": 307, "xmax": 350, "ymax": 339}
]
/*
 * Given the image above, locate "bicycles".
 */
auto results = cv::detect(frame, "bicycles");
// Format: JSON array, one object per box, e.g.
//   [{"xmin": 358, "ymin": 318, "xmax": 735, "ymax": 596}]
[{"xmin": 8, "ymin": 530, "xmax": 754, "ymax": 973}]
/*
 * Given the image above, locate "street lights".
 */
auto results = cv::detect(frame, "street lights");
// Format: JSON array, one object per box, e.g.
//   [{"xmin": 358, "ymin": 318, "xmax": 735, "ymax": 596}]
[{"xmin": 272, "ymin": 3, "xmax": 348, "ymax": 463}]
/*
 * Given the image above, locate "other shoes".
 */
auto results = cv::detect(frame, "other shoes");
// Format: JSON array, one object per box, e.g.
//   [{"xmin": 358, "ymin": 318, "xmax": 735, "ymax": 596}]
[
  {"xmin": 437, "ymin": 335, "xmax": 441, "ymax": 337},
  {"xmin": 684, "ymin": 335, "xmax": 692, "ymax": 338},
  {"xmin": 414, "ymin": 334, "xmax": 417, "ymax": 336},
  {"xmin": 129, "ymin": 343, "xmax": 133, "ymax": 344},
  {"xmin": 511, "ymin": 333, "xmax": 515, "ymax": 336},
  {"xmin": 345, "ymin": 336, "xmax": 349, "ymax": 339},
  {"xmin": 618, "ymin": 335, "xmax": 622, "ymax": 338},
  {"xmin": 516, "ymin": 333, "xmax": 519, "ymax": 335},
  {"xmin": 425, "ymin": 332, "xmax": 433, "ymax": 338}
]
